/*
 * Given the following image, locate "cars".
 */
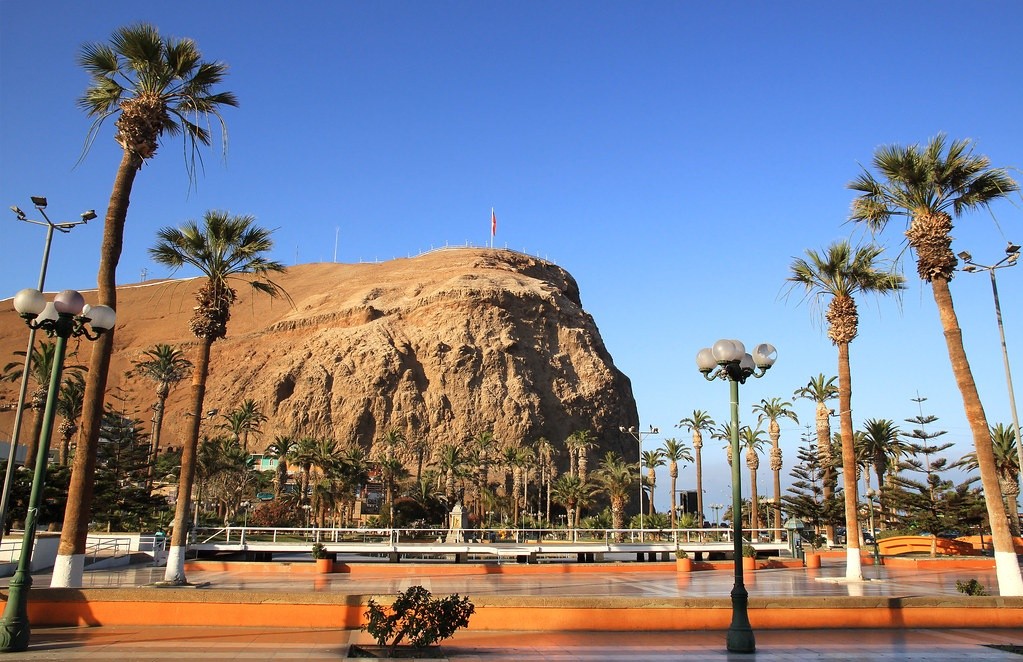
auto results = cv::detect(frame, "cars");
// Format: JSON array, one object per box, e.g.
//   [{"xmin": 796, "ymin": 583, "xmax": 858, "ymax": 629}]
[
  {"xmin": 722, "ymin": 532, "xmax": 734, "ymax": 540},
  {"xmin": 803, "ymin": 527, "xmax": 881, "ymax": 544},
  {"xmin": 782, "ymin": 531, "xmax": 788, "ymax": 542},
  {"xmin": 915, "ymin": 529, "xmax": 958, "ymax": 540},
  {"xmin": 757, "ymin": 532, "xmax": 772, "ymax": 540}
]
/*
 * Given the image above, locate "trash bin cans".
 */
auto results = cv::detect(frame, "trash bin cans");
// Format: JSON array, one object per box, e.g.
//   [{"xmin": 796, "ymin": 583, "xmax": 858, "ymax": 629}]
[{"xmin": 795, "ymin": 540, "xmax": 804, "ymax": 559}]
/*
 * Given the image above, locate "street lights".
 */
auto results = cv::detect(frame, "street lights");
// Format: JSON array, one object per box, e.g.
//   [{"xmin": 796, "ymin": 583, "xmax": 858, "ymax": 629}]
[
  {"xmin": 239, "ymin": 500, "xmax": 255, "ymax": 528},
  {"xmin": 674, "ymin": 504, "xmax": 685, "ymax": 542},
  {"xmin": 859, "ymin": 487, "xmax": 884, "ymax": 567},
  {"xmin": 617, "ymin": 426, "xmax": 662, "ymax": 544},
  {"xmin": 302, "ymin": 503, "xmax": 313, "ymax": 541},
  {"xmin": 559, "ymin": 515, "xmax": 568, "ymax": 539},
  {"xmin": 0, "ymin": 290, "xmax": 117, "ymax": 652},
  {"xmin": 956, "ymin": 243, "xmax": 1022, "ymax": 479},
  {"xmin": 534, "ymin": 512, "xmax": 545, "ymax": 540},
  {"xmin": 694, "ymin": 338, "xmax": 779, "ymax": 655},
  {"xmin": 485, "ymin": 511, "xmax": 496, "ymax": 543},
  {"xmin": 0, "ymin": 192, "xmax": 98, "ymax": 539},
  {"xmin": 520, "ymin": 510, "xmax": 530, "ymax": 543},
  {"xmin": 709, "ymin": 502, "xmax": 725, "ymax": 544}
]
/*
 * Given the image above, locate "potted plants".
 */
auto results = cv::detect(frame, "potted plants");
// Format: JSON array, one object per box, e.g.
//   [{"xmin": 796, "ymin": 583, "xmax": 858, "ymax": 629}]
[
  {"xmin": 806, "ymin": 534, "xmax": 826, "ymax": 569},
  {"xmin": 742, "ymin": 545, "xmax": 757, "ymax": 570},
  {"xmin": 675, "ymin": 549, "xmax": 691, "ymax": 572},
  {"xmin": 312, "ymin": 542, "xmax": 334, "ymax": 574}
]
